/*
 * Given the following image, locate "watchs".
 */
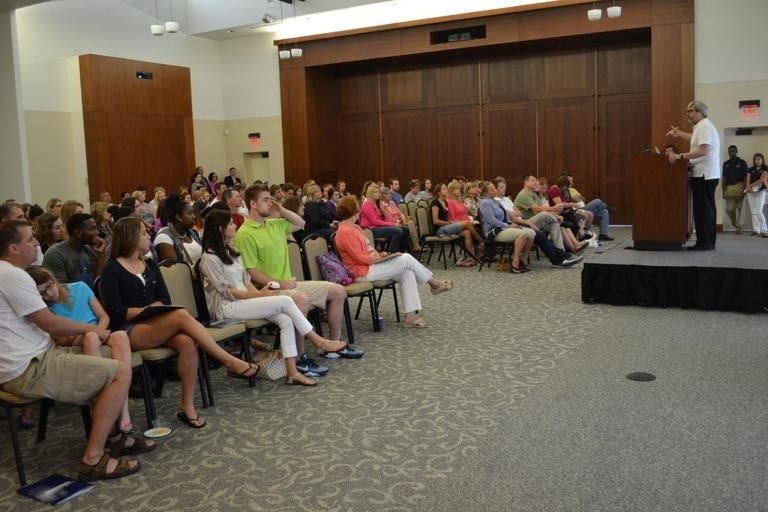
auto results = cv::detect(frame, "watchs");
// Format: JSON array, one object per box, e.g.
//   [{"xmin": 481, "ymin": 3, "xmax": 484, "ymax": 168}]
[{"xmin": 680, "ymin": 152, "xmax": 684, "ymax": 159}]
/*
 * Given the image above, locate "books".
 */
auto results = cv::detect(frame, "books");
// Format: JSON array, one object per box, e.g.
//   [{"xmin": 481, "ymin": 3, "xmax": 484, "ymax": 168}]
[{"xmin": 18, "ymin": 474, "xmax": 96, "ymax": 505}]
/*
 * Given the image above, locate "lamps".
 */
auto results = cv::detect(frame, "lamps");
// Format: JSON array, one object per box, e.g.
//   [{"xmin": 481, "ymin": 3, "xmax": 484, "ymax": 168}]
[
  {"xmin": 291, "ymin": 1, "xmax": 302, "ymax": 58},
  {"xmin": 150, "ymin": 3, "xmax": 164, "ymax": 35},
  {"xmin": 607, "ymin": 2, "xmax": 621, "ymax": 19},
  {"xmin": 165, "ymin": 2, "xmax": 179, "ymax": 34},
  {"xmin": 588, "ymin": 1, "xmax": 602, "ymax": 22},
  {"xmin": 279, "ymin": 3, "xmax": 290, "ymax": 59}
]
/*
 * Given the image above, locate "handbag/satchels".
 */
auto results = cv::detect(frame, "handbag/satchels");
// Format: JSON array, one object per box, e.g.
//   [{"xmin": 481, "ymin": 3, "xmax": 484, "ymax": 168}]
[{"xmin": 315, "ymin": 251, "xmax": 354, "ymax": 286}]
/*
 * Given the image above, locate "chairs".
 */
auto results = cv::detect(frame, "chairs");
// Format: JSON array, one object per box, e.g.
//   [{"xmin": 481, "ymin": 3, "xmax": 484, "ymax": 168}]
[
  {"xmin": 79, "ymin": 350, "xmax": 159, "ymax": 442},
  {"xmin": 0, "ymin": 379, "xmax": 103, "ymax": 486},
  {"xmin": 374, "ymin": 233, "xmax": 390, "ymax": 252},
  {"xmin": 93, "ymin": 271, "xmax": 209, "ymax": 419},
  {"xmin": 302, "ymin": 233, "xmax": 381, "ymax": 334},
  {"xmin": 414, "ymin": 206, "xmax": 457, "ymax": 271},
  {"xmin": 479, "ymin": 240, "xmax": 521, "ymax": 273},
  {"xmin": 286, "ymin": 241, "xmax": 355, "ymax": 346},
  {"xmin": 330, "ymin": 229, "xmax": 400, "ymax": 323},
  {"xmin": 400, "ymin": 198, "xmax": 444, "ymax": 220},
  {"xmin": 194, "ymin": 255, "xmax": 303, "ymax": 386},
  {"xmin": 156, "ymin": 258, "xmax": 256, "ymax": 405}
]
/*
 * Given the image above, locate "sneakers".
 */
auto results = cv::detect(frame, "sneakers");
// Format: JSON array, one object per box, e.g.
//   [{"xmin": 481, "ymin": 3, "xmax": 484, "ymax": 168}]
[
  {"xmin": 762, "ymin": 233, "xmax": 768, "ymax": 237},
  {"xmin": 575, "ymin": 240, "xmax": 589, "ymax": 250},
  {"xmin": 608, "ymin": 207, "xmax": 617, "ymax": 213},
  {"xmin": 736, "ymin": 230, "xmax": 741, "ymax": 234},
  {"xmin": 561, "ymin": 255, "xmax": 584, "ymax": 265},
  {"xmin": 598, "ymin": 234, "xmax": 614, "ymax": 241},
  {"xmin": 413, "ymin": 247, "xmax": 427, "ymax": 253},
  {"xmin": 323, "ymin": 345, "xmax": 365, "ymax": 358},
  {"xmin": 750, "ymin": 232, "xmax": 760, "ymax": 237},
  {"xmin": 574, "ymin": 249, "xmax": 585, "ymax": 256},
  {"xmin": 295, "ymin": 353, "xmax": 329, "ymax": 377}
]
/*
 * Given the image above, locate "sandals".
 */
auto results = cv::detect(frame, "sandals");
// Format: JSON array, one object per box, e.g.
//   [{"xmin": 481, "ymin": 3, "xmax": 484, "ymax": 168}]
[
  {"xmin": 285, "ymin": 372, "xmax": 317, "ymax": 386},
  {"xmin": 315, "ymin": 340, "xmax": 347, "ymax": 358},
  {"xmin": 105, "ymin": 430, "xmax": 156, "ymax": 456},
  {"xmin": 402, "ymin": 318, "xmax": 428, "ymax": 329},
  {"xmin": 79, "ymin": 453, "xmax": 140, "ymax": 481},
  {"xmin": 431, "ymin": 279, "xmax": 454, "ymax": 295}
]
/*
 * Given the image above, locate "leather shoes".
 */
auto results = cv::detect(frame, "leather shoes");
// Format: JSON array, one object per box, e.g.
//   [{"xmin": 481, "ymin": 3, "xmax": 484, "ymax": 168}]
[{"xmin": 686, "ymin": 243, "xmax": 716, "ymax": 251}]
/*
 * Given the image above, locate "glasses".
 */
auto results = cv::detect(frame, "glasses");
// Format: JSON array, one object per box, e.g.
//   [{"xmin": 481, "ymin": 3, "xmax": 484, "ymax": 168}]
[{"xmin": 40, "ymin": 282, "xmax": 54, "ymax": 296}]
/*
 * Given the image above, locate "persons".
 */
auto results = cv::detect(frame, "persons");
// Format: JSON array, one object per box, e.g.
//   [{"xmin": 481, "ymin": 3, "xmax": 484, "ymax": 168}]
[
  {"xmin": 548, "ymin": 177, "xmax": 593, "ymax": 241},
  {"xmin": 200, "ymin": 209, "xmax": 347, "ymax": 386},
  {"xmin": 26, "ymin": 266, "xmax": 131, "ymax": 435},
  {"xmin": 335, "ymin": 196, "xmax": 454, "ymax": 328},
  {"xmin": 665, "ymin": 145, "xmax": 675, "ymax": 155},
  {"xmin": 535, "ymin": 179, "xmax": 590, "ymax": 255},
  {"xmin": 448, "ymin": 176, "xmax": 457, "ymax": 184},
  {"xmin": 421, "ymin": 179, "xmax": 433, "ymax": 199},
  {"xmin": 376, "ymin": 182, "xmax": 384, "ymax": 193},
  {"xmin": 539, "ymin": 177, "xmax": 564, "ymax": 223},
  {"xmin": 0, "ymin": 203, "xmax": 43, "ymax": 268},
  {"xmin": 41, "ymin": 214, "xmax": 105, "ymax": 284},
  {"xmin": 458, "ymin": 176, "xmax": 467, "ymax": 190},
  {"xmin": 464, "ymin": 182, "xmax": 480, "ymax": 218},
  {"xmin": 666, "ymin": 100, "xmax": 721, "ymax": 251},
  {"xmin": 563, "ymin": 188, "xmax": 593, "ymax": 228},
  {"xmin": 749, "ymin": 153, "xmax": 768, "ymax": 238},
  {"xmin": 389, "ymin": 177, "xmax": 402, "ymax": 204},
  {"xmin": 28, "ymin": 205, "xmax": 44, "ymax": 225},
  {"xmin": 91, "ymin": 166, "xmax": 246, "ymax": 224},
  {"xmin": 480, "ymin": 182, "xmax": 536, "ymax": 273},
  {"xmin": 337, "ymin": 182, "xmax": 349, "ymax": 198},
  {"xmin": 1, "ymin": 220, "xmax": 156, "ymax": 482},
  {"xmin": 36, "ymin": 215, "xmax": 67, "ymax": 252},
  {"xmin": 405, "ymin": 182, "xmax": 421, "ymax": 203},
  {"xmin": 61, "ymin": 200, "xmax": 84, "ymax": 220},
  {"xmin": 493, "ymin": 177, "xmax": 583, "ymax": 268},
  {"xmin": 321, "ymin": 187, "xmax": 331, "ymax": 202},
  {"xmin": 326, "ymin": 188, "xmax": 343, "ymax": 221},
  {"xmin": 722, "ymin": 145, "xmax": 748, "ymax": 234},
  {"xmin": 233, "ymin": 184, "xmax": 364, "ymax": 377},
  {"xmin": 447, "ymin": 182, "xmax": 469, "ymax": 222},
  {"xmin": 380, "ymin": 189, "xmax": 427, "ymax": 253},
  {"xmin": 361, "ymin": 186, "xmax": 409, "ymax": 253},
  {"xmin": 514, "ymin": 175, "xmax": 564, "ymax": 251},
  {"xmin": 567, "ymin": 176, "xmax": 614, "ymax": 240},
  {"xmin": 21, "ymin": 204, "xmax": 32, "ymax": 218},
  {"xmin": 281, "ymin": 196, "xmax": 329, "ymax": 324},
  {"xmin": 362, "ymin": 180, "xmax": 384, "ymax": 215},
  {"xmin": 99, "ymin": 219, "xmax": 260, "ymax": 428},
  {"xmin": 304, "ymin": 185, "xmax": 338, "ymax": 239},
  {"xmin": 430, "ymin": 183, "xmax": 482, "ymax": 260},
  {"xmin": 153, "ymin": 202, "xmax": 202, "ymax": 278},
  {"xmin": 47, "ymin": 198, "xmax": 63, "ymax": 216}
]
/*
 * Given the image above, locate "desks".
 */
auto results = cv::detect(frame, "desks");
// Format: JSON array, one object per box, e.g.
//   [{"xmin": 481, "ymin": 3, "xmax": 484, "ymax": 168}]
[{"xmin": 626, "ymin": 148, "xmax": 690, "ymax": 251}]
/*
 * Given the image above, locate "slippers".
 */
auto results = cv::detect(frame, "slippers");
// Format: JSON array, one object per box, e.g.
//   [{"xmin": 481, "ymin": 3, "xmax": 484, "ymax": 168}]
[
  {"xmin": 18, "ymin": 413, "xmax": 36, "ymax": 429},
  {"xmin": 511, "ymin": 260, "xmax": 520, "ymax": 273},
  {"xmin": 177, "ymin": 411, "xmax": 207, "ymax": 428},
  {"xmin": 521, "ymin": 261, "xmax": 528, "ymax": 271},
  {"xmin": 227, "ymin": 363, "xmax": 261, "ymax": 379}
]
